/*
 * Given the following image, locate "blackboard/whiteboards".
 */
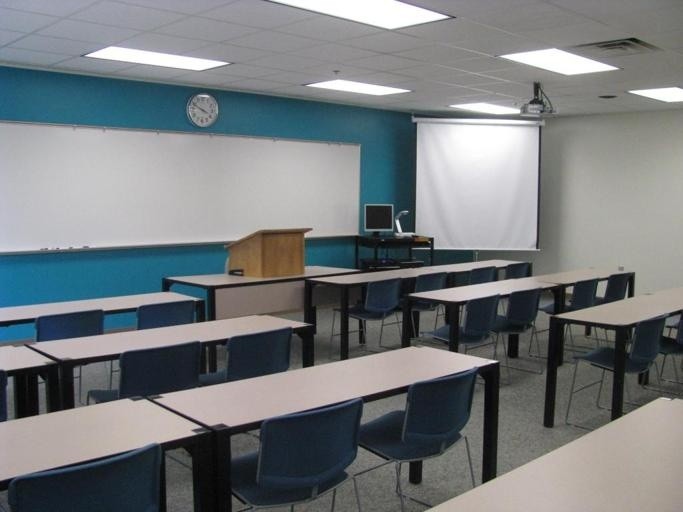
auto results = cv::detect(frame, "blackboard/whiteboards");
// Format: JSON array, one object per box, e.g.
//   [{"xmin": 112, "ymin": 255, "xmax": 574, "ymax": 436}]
[{"xmin": 0, "ymin": 120, "xmax": 361, "ymax": 258}]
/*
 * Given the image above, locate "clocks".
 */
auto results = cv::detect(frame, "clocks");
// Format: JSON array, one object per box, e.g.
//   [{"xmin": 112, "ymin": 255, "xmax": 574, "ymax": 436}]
[{"xmin": 187, "ymin": 93, "xmax": 219, "ymax": 129}]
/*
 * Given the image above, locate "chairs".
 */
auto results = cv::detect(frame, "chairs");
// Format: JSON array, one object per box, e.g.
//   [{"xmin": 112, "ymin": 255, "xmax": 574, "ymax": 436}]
[
  {"xmin": 135, "ymin": 299, "xmax": 195, "ymax": 328},
  {"xmin": 88, "ymin": 340, "xmax": 203, "ymax": 407},
  {"xmin": 232, "ymin": 399, "xmax": 366, "ymax": 512},
  {"xmin": 505, "ymin": 261, "xmax": 532, "ymax": 279},
  {"xmin": 597, "ymin": 272, "xmax": 632, "ymax": 306},
  {"xmin": 329, "ymin": 278, "xmax": 403, "ymax": 348},
  {"xmin": 467, "ymin": 266, "xmax": 498, "ymax": 285},
  {"xmin": 567, "ymin": 313, "xmax": 661, "ymax": 423},
  {"xmin": 661, "ymin": 310, "xmax": 683, "ymax": 358},
  {"xmin": 359, "ymin": 366, "xmax": 476, "ymax": 510},
  {"xmin": 6, "ymin": 441, "xmax": 169, "ymax": 512},
  {"xmin": 200, "ymin": 327, "xmax": 293, "ymax": 388},
  {"xmin": 541, "ymin": 277, "xmax": 599, "ymax": 348},
  {"xmin": 36, "ymin": 309, "xmax": 105, "ymax": 339},
  {"xmin": 401, "ymin": 271, "xmax": 447, "ymax": 339},
  {"xmin": 482, "ymin": 287, "xmax": 540, "ymax": 371}
]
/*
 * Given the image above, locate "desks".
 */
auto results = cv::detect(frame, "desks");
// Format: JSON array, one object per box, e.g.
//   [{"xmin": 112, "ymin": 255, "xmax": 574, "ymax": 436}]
[
  {"xmin": 147, "ymin": 345, "xmax": 502, "ymax": 512},
  {"xmin": 302, "ymin": 268, "xmax": 432, "ymax": 341},
  {"xmin": 0, "ymin": 396, "xmax": 213, "ymax": 512},
  {"xmin": 159, "ymin": 265, "xmax": 360, "ymax": 323},
  {"xmin": 1, "ymin": 344, "xmax": 58, "ymax": 413},
  {"xmin": 421, "ymin": 258, "xmax": 528, "ymax": 295},
  {"xmin": 356, "ymin": 236, "xmax": 434, "ymax": 272},
  {"xmin": 409, "ymin": 280, "xmax": 561, "ymax": 368},
  {"xmin": 540, "ymin": 290, "xmax": 683, "ymax": 425},
  {"xmin": 1, "ymin": 291, "xmax": 206, "ymax": 333},
  {"xmin": 33, "ymin": 314, "xmax": 315, "ymax": 412},
  {"xmin": 525, "ymin": 265, "xmax": 634, "ymax": 337}
]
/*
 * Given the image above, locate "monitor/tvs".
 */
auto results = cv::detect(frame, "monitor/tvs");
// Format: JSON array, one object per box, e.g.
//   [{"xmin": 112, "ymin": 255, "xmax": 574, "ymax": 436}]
[{"xmin": 364, "ymin": 203, "xmax": 396, "ymax": 236}]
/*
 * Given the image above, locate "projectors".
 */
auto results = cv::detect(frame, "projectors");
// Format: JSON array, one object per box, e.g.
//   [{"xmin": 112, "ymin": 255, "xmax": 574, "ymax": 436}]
[{"xmin": 519, "ymin": 101, "xmax": 555, "ymax": 117}]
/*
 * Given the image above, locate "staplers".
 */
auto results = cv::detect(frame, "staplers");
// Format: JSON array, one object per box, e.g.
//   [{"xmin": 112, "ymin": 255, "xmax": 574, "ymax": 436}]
[{"xmin": 229, "ymin": 269, "xmax": 244, "ymax": 276}]
